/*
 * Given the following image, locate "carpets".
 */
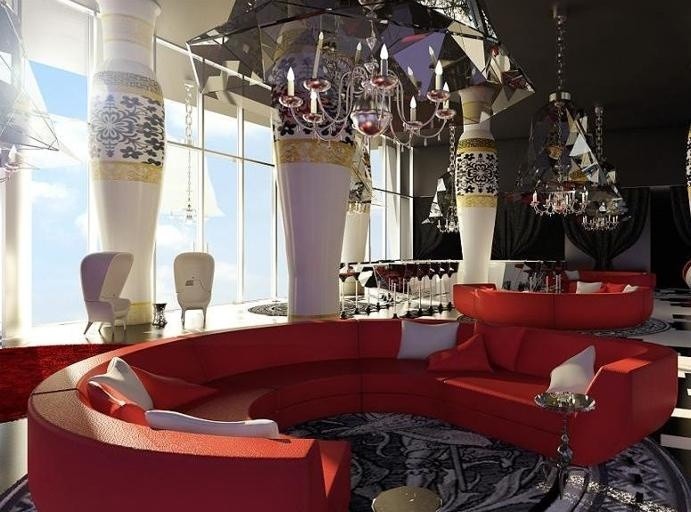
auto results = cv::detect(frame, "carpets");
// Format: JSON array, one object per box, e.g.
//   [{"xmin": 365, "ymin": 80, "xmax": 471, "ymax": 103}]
[
  {"xmin": 457, "ymin": 316, "xmax": 670, "ymax": 336},
  {"xmin": 248, "ymin": 302, "xmax": 375, "ymax": 316},
  {"xmin": 0, "ymin": 412, "xmax": 690, "ymax": 511},
  {"xmin": 0, "ymin": 344, "xmax": 133, "ymax": 422}
]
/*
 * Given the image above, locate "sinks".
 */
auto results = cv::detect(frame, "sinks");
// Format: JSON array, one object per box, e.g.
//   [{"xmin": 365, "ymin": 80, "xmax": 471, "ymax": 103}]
[
  {"xmin": 28, "ymin": 319, "xmax": 678, "ymax": 511},
  {"xmin": 564, "ymin": 270, "xmax": 657, "ymax": 293},
  {"xmin": 453, "ymin": 284, "xmax": 653, "ymax": 329}
]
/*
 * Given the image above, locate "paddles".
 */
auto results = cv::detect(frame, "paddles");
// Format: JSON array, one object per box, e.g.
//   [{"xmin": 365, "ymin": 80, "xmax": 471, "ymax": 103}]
[
  {"xmin": 248, "ymin": 302, "xmax": 375, "ymax": 316},
  {"xmin": 457, "ymin": 316, "xmax": 670, "ymax": 336},
  {"xmin": 0, "ymin": 344, "xmax": 133, "ymax": 422},
  {"xmin": 0, "ymin": 412, "xmax": 690, "ymax": 511}
]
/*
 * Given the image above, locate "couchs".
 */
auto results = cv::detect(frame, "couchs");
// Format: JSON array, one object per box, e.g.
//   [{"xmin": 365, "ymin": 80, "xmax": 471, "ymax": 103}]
[
  {"xmin": 28, "ymin": 319, "xmax": 678, "ymax": 511},
  {"xmin": 564, "ymin": 270, "xmax": 657, "ymax": 293},
  {"xmin": 453, "ymin": 284, "xmax": 653, "ymax": 329}
]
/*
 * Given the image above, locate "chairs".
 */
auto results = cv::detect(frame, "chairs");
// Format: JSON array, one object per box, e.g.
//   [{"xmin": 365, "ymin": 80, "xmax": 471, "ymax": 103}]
[
  {"xmin": 174, "ymin": 253, "xmax": 214, "ymax": 328},
  {"xmin": 82, "ymin": 252, "xmax": 134, "ymax": 340},
  {"xmin": 339, "ymin": 259, "xmax": 458, "ymax": 319}
]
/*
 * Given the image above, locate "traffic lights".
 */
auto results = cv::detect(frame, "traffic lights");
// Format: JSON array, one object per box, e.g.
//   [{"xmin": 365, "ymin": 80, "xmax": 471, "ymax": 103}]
[
  {"xmin": 576, "ymin": 281, "xmax": 639, "ymax": 294},
  {"xmin": 397, "ymin": 319, "xmax": 525, "ymax": 373},
  {"xmin": 87, "ymin": 357, "xmax": 279, "ymax": 438},
  {"xmin": 545, "ymin": 345, "xmax": 596, "ymax": 394}
]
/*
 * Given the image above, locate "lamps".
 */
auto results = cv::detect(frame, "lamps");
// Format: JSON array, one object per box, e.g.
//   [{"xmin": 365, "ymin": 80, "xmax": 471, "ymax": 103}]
[
  {"xmin": 0, "ymin": 0, "xmax": 60, "ymax": 183},
  {"xmin": 518, "ymin": 1, "xmax": 591, "ymax": 218},
  {"xmin": 279, "ymin": 0, "xmax": 456, "ymax": 150},
  {"xmin": 581, "ymin": 105, "xmax": 630, "ymax": 230},
  {"xmin": 421, "ymin": 106, "xmax": 462, "ymax": 230},
  {"xmin": 82, "ymin": 252, "xmax": 134, "ymax": 340},
  {"xmin": 174, "ymin": 253, "xmax": 214, "ymax": 328},
  {"xmin": 339, "ymin": 259, "xmax": 458, "ymax": 319}
]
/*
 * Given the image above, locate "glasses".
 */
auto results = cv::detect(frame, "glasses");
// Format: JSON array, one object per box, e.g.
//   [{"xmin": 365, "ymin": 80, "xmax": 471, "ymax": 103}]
[{"xmin": 0, "ymin": 0, "xmax": 60, "ymax": 183}]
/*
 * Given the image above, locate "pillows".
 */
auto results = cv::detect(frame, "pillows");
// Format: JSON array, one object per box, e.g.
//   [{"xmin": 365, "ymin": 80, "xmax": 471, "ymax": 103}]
[
  {"xmin": 87, "ymin": 357, "xmax": 279, "ymax": 438},
  {"xmin": 576, "ymin": 281, "xmax": 639, "ymax": 294},
  {"xmin": 397, "ymin": 319, "xmax": 525, "ymax": 373},
  {"xmin": 545, "ymin": 345, "xmax": 596, "ymax": 394}
]
